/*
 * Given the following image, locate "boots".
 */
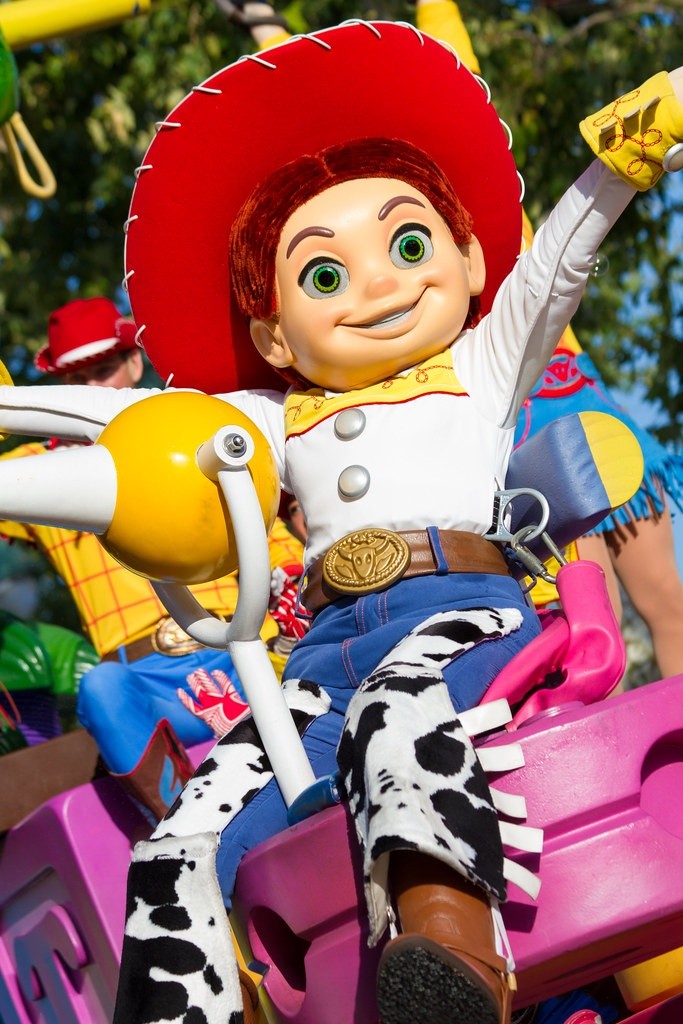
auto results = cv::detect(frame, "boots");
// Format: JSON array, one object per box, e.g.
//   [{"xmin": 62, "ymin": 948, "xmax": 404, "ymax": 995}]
[
  {"xmin": 111, "ymin": 718, "xmax": 197, "ymax": 825},
  {"xmin": 374, "ymin": 849, "xmax": 517, "ymax": 1024}
]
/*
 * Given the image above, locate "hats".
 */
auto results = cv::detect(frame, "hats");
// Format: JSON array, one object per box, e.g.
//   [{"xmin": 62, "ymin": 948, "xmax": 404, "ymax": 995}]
[{"xmin": 34, "ymin": 297, "xmax": 143, "ymax": 375}]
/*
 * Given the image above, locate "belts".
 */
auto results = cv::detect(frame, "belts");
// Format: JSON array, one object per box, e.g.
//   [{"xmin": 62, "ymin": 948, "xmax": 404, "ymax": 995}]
[
  {"xmin": 299, "ymin": 528, "xmax": 511, "ymax": 614},
  {"xmin": 101, "ymin": 612, "xmax": 235, "ymax": 668}
]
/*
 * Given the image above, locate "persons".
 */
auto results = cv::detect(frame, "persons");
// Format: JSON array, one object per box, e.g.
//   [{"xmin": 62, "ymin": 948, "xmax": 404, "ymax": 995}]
[
  {"xmin": 1, "ymin": 24, "xmax": 683, "ymax": 1024},
  {"xmin": 0, "ymin": 297, "xmax": 309, "ymax": 822},
  {"xmin": 236, "ymin": 1, "xmax": 683, "ymax": 693}
]
